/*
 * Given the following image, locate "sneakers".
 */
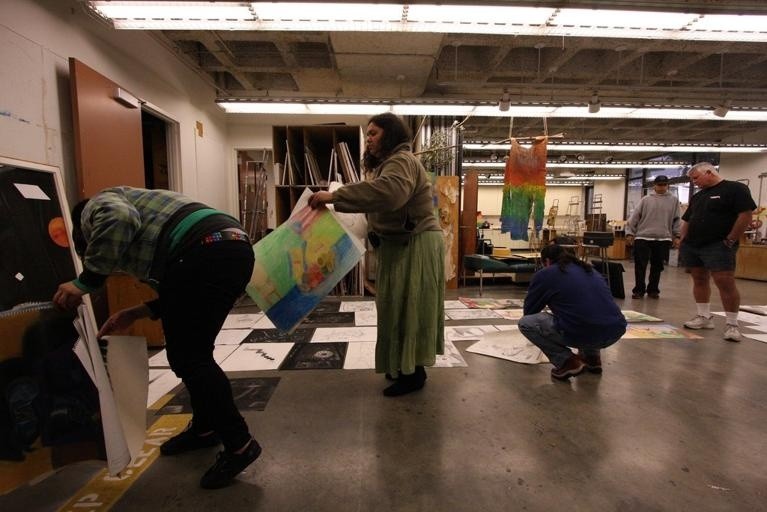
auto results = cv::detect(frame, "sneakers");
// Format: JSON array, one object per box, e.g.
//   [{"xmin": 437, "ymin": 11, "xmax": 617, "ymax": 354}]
[
  {"xmin": 683, "ymin": 314, "xmax": 715, "ymax": 329},
  {"xmin": 723, "ymin": 323, "xmax": 742, "ymax": 342},
  {"xmin": 631, "ymin": 284, "xmax": 660, "ymax": 300},
  {"xmin": 577, "ymin": 351, "xmax": 603, "ymax": 374},
  {"xmin": 200, "ymin": 438, "xmax": 261, "ymax": 490},
  {"xmin": 550, "ymin": 354, "xmax": 589, "ymax": 380},
  {"xmin": 160, "ymin": 421, "xmax": 222, "ymax": 456}
]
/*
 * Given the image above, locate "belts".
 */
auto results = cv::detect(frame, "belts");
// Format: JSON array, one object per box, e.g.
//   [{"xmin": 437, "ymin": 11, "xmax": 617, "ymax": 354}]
[{"xmin": 200, "ymin": 232, "xmax": 249, "ymax": 245}]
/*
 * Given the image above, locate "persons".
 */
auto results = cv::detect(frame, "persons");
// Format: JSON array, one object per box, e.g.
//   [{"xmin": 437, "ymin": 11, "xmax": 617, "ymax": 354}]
[
  {"xmin": 51, "ymin": 185, "xmax": 263, "ymax": 496},
  {"xmin": 676, "ymin": 161, "xmax": 756, "ymax": 342},
  {"xmin": 308, "ymin": 114, "xmax": 447, "ymax": 398},
  {"xmin": 625, "ymin": 175, "xmax": 683, "ymax": 299},
  {"xmin": 517, "ymin": 243, "xmax": 627, "ymax": 382}
]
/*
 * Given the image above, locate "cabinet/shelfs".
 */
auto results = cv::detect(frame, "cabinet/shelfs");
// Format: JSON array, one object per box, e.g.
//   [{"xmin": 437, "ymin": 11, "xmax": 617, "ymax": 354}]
[{"xmin": 270, "ymin": 124, "xmax": 363, "ymax": 299}]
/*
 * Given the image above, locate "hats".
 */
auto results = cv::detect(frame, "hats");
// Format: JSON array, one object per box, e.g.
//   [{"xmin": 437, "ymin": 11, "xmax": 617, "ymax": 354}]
[{"xmin": 656, "ymin": 175, "xmax": 670, "ymax": 183}]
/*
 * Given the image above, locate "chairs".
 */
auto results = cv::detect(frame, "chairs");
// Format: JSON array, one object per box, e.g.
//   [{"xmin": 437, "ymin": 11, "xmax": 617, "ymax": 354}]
[{"xmin": 463, "ymin": 253, "xmax": 538, "ymax": 298}]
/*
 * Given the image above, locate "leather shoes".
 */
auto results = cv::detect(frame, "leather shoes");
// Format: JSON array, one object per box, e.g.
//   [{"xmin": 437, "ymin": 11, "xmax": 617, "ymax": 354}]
[{"xmin": 384, "ymin": 367, "xmax": 426, "ymax": 396}]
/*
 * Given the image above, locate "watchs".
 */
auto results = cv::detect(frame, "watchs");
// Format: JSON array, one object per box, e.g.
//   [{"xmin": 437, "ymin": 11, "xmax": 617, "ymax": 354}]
[{"xmin": 724, "ymin": 237, "xmax": 735, "ymax": 245}]
